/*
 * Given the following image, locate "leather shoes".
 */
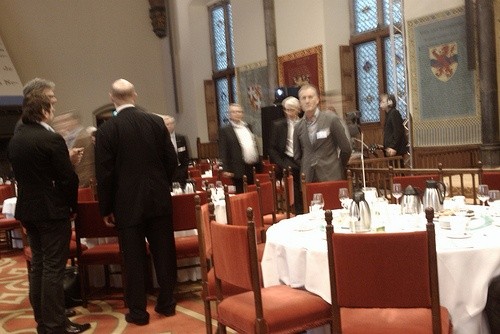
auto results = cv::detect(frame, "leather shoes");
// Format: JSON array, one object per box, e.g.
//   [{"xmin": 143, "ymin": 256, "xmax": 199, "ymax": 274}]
[
  {"xmin": 37, "ymin": 320, "xmax": 91, "ymax": 334},
  {"xmin": 64, "ymin": 310, "xmax": 77, "ymax": 317}
]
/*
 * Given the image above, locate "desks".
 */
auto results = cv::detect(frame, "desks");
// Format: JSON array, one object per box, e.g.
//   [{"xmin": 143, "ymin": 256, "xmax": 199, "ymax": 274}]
[
  {"xmin": 260, "ymin": 203, "xmax": 500, "ymax": 334},
  {"xmin": 76, "ymin": 190, "xmax": 234, "ymax": 287},
  {"xmin": 2, "ymin": 196, "xmax": 22, "ymax": 248}
]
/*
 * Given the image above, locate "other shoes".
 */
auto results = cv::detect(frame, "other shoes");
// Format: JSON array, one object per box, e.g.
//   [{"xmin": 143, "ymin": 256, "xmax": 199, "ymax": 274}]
[
  {"xmin": 154, "ymin": 304, "xmax": 175, "ymax": 317},
  {"xmin": 125, "ymin": 312, "xmax": 149, "ymax": 325}
]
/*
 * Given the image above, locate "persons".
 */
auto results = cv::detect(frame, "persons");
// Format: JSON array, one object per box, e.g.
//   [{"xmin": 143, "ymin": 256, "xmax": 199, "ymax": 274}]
[
  {"xmin": 164, "ymin": 116, "xmax": 189, "ymax": 188},
  {"xmin": 55, "ymin": 104, "xmax": 116, "ymax": 187},
  {"xmin": 379, "ymin": 93, "xmax": 410, "ymax": 177},
  {"xmin": 219, "ymin": 103, "xmax": 260, "ymax": 195},
  {"xmin": 8, "ymin": 87, "xmax": 91, "ymax": 334},
  {"xmin": 270, "ymin": 96, "xmax": 304, "ymax": 216},
  {"xmin": 13, "ymin": 77, "xmax": 76, "ymax": 323},
  {"xmin": 326, "ymin": 106, "xmax": 362, "ymax": 154},
  {"xmin": 94, "ymin": 78, "xmax": 178, "ymax": 326},
  {"xmin": 293, "ymin": 85, "xmax": 351, "ymax": 183}
]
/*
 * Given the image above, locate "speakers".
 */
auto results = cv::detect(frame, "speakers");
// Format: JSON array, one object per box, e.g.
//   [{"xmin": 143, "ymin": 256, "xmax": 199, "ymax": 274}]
[{"xmin": 260, "ymin": 104, "xmax": 304, "ymax": 160}]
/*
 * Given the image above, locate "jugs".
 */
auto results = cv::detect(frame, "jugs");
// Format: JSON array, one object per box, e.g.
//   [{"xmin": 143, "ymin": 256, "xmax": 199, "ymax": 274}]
[
  {"xmin": 400, "ymin": 184, "xmax": 422, "ymax": 215},
  {"xmin": 349, "ymin": 191, "xmax": 371, "ymax": 232},
  {"xmin": 422, "ymin": 179, "xmax": 446, "ymax": 213},
  {"xmin": 185, "ymin": 179, "xmax": 196, "ymax": 194}
]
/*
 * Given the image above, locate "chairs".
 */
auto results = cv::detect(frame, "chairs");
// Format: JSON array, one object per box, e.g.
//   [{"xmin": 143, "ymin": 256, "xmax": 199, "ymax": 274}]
[{"xmin": 0, "ymin": 158, "xmax": 500, "ymax": 334}]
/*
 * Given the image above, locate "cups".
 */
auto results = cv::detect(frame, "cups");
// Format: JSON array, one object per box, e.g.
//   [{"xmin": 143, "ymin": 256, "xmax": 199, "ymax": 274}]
[
  {"xmin": 216, "ymin": 180, "xmax": 225, "ymax": 197},
  {"xmin": 449, "ymin": 216, "xmax": 470, "ymax": 236},
  {"xmin": 171, "ymin": 182, "xmax": 181, "ymax": 196},
  {"xmin": 310, "ymin": 200, "xmax": 320, "ymax": 220},
  {"xmin": 207, "ymin": 183, "xmax": 217, "ymax": 197}
]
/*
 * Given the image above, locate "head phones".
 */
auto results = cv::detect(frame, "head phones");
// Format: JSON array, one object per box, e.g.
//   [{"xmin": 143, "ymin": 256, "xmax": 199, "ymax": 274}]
[{"xmin": 387, "ymin": 93, "xmax": 393, "ymax": 107}]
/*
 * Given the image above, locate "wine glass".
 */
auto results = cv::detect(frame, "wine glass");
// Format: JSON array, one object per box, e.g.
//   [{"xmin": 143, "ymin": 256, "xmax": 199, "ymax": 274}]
[
  {"xmin": 313, "ymin": 193, "xmax": 325, "ymax": 212},
  {"xmin": 392, "ymin": 183, "xmax": 403, "ymax": 210},
  {"xmin": 476, "ymin": 186, "xmax": 500, "ymax": 219}
]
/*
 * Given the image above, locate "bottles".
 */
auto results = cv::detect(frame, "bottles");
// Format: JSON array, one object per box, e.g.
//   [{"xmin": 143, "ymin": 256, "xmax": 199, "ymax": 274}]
[{"xmin": 338, "ymin": 188, "xmax": 349, "ymax": 206}]
